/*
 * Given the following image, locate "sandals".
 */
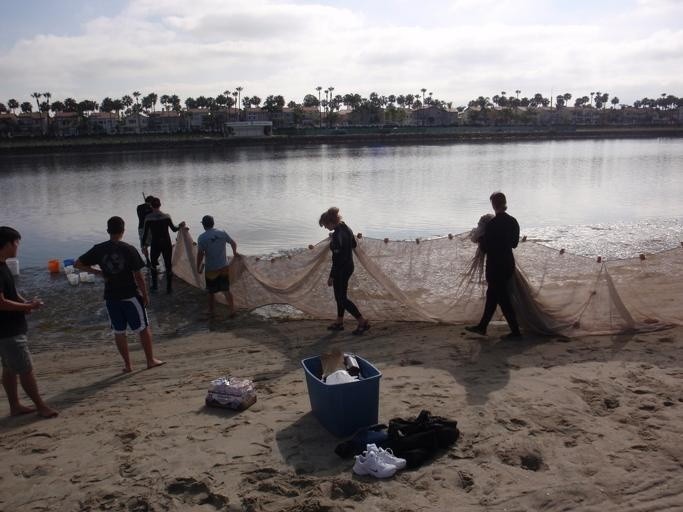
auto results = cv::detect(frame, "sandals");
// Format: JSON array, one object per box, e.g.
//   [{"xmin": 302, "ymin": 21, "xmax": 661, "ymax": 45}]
[
  {"xmin": 328, "ymin": 322, "xmax": 344, "ymax": 330},
  {"xmin": 352, "ymin": 321, "xmax": 371, "ymax": 335}
]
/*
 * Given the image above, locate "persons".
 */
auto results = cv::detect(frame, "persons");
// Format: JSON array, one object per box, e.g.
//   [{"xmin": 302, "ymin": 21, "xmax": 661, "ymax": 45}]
[
  {"xmin": 141, "ymin": 198, "xmax": 184, "ymax": 293},
  {"xmin": 74, "ymin": 215, "xmax": 166, "ymax": 370},
  {"xmin": 137, "ymin": 196, "xmax": 160, "ymax": 267},
  {"xmin": 318, "ymin": 207, "xmax": 371, "ymax": 335},
  {"xmin": 0, "ymin": 226, "xmax": 60, "ymax": 417},
  {"xmin": 196, "ymin": 215, "xmax": 240, "ymax": 317},
  {"xmin": 465, "ymin": 193, "xmax": 522, "ymax": 342}
]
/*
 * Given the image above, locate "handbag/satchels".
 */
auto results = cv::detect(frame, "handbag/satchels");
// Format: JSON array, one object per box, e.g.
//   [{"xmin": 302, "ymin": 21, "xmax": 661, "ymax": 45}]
[{"xmin": 335, "ymin": 409, "xmax": 460, "ymax": 471}]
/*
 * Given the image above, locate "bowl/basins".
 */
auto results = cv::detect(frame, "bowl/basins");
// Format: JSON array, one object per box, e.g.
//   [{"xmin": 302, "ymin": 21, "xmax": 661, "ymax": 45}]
[{"xmin": 325, "ymin": 369, "xmax": 355, "ymax": 385}]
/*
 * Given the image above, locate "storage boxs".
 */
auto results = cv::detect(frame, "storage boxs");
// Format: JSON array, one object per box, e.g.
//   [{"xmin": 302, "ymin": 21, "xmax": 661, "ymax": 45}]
[{"xmin": 301, "ymin": 352, "xmax": 382, "ymax": 439}]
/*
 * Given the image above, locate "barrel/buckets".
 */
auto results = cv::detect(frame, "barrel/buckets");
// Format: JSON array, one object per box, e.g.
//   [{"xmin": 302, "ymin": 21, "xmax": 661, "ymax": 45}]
[
  {"xmin": 67, "ymin": 274, "xmax": 80, "ymax": 285},
  {"xmin": 5, "ymin": 259, "xmax": 20, "ymax": 276},
  {"xmin": 47, "ymin": 259, "xmax": 60, "ymax": 273},
  {"xmin": 79, "ymin": 272, "xmax": 88, "ymax": 283},
  {"xmin": 64, "ymin": 266, "xmax": 76, "ymax": 275},
  {"xmin": 88, "ymin": 274, "xmax": 95, "ymax": 283},
  {"xmin": 63, "ymin": 260, "xmax": 75, "ymax": 266}
]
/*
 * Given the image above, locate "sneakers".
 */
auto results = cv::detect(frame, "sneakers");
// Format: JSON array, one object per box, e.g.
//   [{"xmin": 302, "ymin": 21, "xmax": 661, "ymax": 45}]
[
  {"xmin": 367, "ymin": 444, "xmax": 407, "ymax": 470},
  {"xmin": 465, "ymin": 325, "xmax": 486, "ymax": 335},
  {"xmin": 353, "ymin": 450, "xmax": 398, "ymax": 477}
]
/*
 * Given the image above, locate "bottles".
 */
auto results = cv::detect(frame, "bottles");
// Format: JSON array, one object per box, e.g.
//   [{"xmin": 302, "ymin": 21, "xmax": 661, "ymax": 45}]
[
  {"xmin": 206, "ymin": 375, "xmax": 256, "ymax": 410},
  {"xmin": 346, "ymin": 355, "xmax": 361, "ymax": 375}
]
